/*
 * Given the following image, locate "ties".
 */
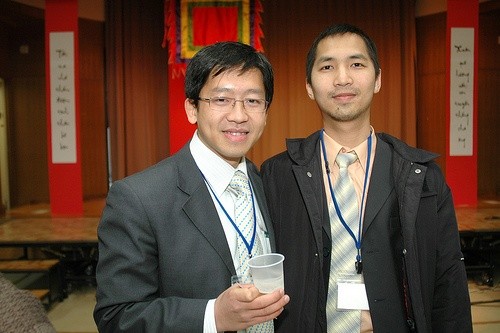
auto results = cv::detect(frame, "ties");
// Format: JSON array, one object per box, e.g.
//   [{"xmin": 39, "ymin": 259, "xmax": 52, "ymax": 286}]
[
  {"xmin": 225, "ymin": 171, "xmax": 274, "ymax": 333},
  {"xmin": 325, "ymin": 152, "xmax": 361, "ymax": 333}
]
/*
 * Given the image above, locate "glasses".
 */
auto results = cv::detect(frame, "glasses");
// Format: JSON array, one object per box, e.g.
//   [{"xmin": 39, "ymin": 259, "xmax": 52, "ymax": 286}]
[{"xmin": 197, "ymin": 96, "xmax": 270, "ymax": 113}]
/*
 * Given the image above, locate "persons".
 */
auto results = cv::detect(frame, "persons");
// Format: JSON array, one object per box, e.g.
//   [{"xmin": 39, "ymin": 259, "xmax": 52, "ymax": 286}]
[
  {"xmin": 258, "ymin": 23, "xmax": 473, "ymax": 332},
  {"xmin": 92, "ymin": 40, "xmax": 290, "ymax": 333}
]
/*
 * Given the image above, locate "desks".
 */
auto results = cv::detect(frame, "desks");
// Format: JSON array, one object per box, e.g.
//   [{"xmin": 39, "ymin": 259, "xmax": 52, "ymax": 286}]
[{"xmin": 0, "ymin": 217, "xmax": 104, "ymax": 289}]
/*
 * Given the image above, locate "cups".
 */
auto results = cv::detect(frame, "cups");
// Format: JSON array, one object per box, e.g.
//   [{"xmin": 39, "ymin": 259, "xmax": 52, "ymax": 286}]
[{"xmin": 246, "ymin": 253, "xmax": 286, "ymax": 297}]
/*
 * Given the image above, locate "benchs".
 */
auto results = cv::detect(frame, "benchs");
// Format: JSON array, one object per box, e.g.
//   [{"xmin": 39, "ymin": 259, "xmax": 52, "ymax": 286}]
[
  {"xmin": 0, "ymin": 260, "xmax": 69, "ymax": 311},
  {"xmin": 29, "ymin": 290, "xmax": 52, "ymax": 311}
]
